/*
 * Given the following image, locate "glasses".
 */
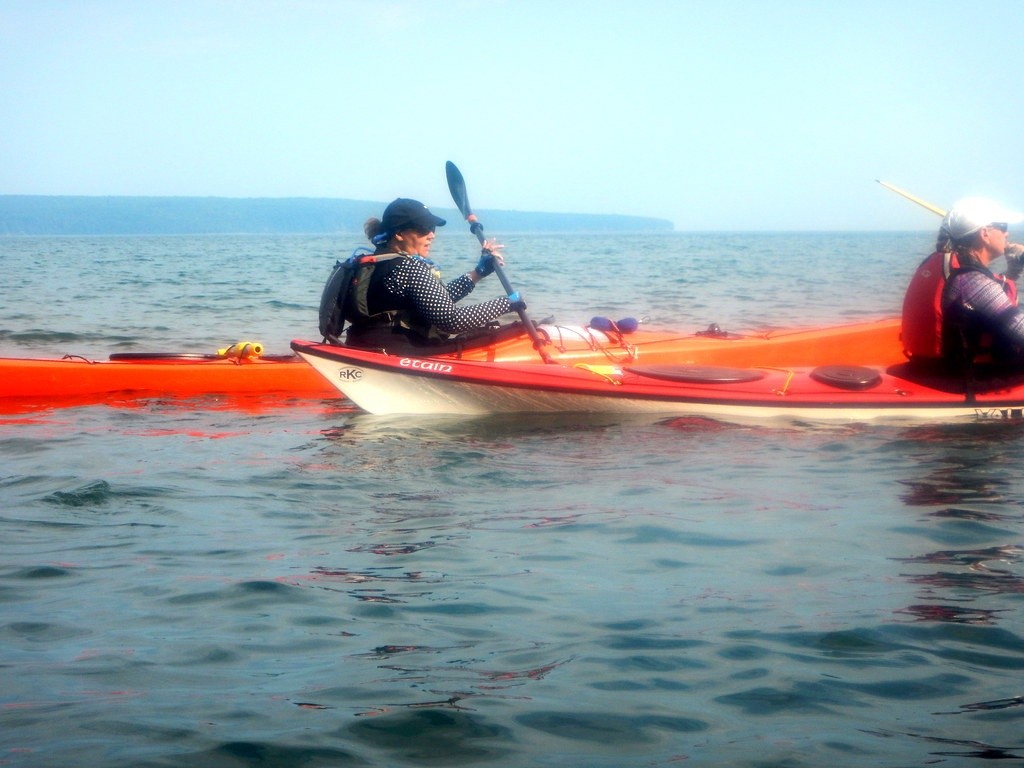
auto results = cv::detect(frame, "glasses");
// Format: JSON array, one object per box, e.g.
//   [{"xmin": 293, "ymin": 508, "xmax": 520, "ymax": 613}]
[
  {"xmin": 406, "ymin": 224, "xmax": 435, "ymax": 235},
  {"xmin": 989, "ymin": 223, "xmax": 1008, "ymax": 232}
]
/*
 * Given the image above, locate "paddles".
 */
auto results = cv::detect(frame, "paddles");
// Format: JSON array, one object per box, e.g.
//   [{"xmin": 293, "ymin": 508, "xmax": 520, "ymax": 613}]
[
  {"xmin": 217, "ymin": 341, "xmax": 264, "ymax": 359},
  {"xmin": 876, "ymin": 179, "xmax": 946, "ymax": 217},
  {"xmin": 446, "ymin": 160, "xmax": 559, "ymax": 364}
]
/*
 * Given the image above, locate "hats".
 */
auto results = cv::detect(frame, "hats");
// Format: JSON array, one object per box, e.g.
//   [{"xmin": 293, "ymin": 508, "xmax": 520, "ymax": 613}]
[
  {"xmin": 941, "ymin": 196, "xmax": 1024, "ymax": 240},
  {"xmin": 382, "ymin": 198, "xmax": 446, "ymax": 232}
]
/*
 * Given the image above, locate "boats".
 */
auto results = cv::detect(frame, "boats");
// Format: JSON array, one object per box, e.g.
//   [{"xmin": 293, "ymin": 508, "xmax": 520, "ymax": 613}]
[
  {"xmin": 1, "ymin": 308, "xmax": 922, "ymax": 422},
  {"xmin": 286, "ymin": 334, "xmax": 1024, "ymax": 428}
]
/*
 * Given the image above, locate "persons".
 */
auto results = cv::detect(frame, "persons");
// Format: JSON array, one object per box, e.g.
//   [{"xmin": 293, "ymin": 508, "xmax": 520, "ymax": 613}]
[
  {"xmin": 899, "ymin": 201, "xmax": 1024, "ymax": 376},
  {"xmin": 345, "ymin": 198, "xmax": 526, "ymax": 359}
]
